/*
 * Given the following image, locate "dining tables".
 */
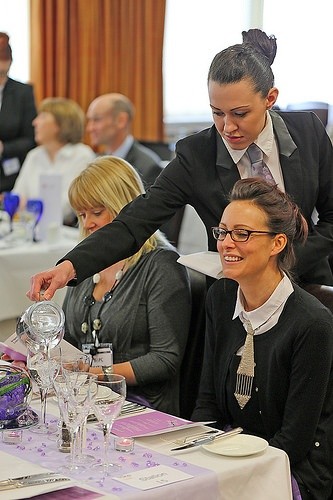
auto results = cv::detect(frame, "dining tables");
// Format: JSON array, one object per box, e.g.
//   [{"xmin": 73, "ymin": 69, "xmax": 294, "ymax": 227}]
[
  {"xmin": 0, "ymin": 219, "xmax": 84, "ymax": 323},
  {"xmin": 0, "ymin": 376, "xmax": 293, "ymax": 500}
]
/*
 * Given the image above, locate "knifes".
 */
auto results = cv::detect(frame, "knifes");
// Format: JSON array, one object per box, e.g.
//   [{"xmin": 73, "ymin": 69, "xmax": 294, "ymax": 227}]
[
  {"xmin": 171, "ymin": 427, "xmax": 243, "ymax": 451},
  {"xmin": 0, "ymin": 471, "xmax": 71, "ymax": 491}
]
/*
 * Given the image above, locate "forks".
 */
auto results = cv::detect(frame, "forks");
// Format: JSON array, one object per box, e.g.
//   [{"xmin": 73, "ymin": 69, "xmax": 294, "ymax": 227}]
[{"xmin": 167, "ymin": 429, "xmax": 221, "ymax": 447}]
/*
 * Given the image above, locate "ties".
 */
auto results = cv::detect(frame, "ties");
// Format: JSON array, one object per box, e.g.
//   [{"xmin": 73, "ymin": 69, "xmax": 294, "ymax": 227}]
[
  {"xmin": 246, "ymin": 143, "xmax": 276, "ymax": 186},
  {"xmin": 234, "ymin": 312, "xmax": 259, "ymax": 410}
]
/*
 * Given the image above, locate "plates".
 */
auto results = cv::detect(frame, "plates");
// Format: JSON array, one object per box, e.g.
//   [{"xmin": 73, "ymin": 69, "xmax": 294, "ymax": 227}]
[
  {"xmin": 201, "ymin": 434, "xmax": 270, "ymax": 457},
  {"xmin": 50, "ymin": 384, "xmax": 113, "ymax": 403}
]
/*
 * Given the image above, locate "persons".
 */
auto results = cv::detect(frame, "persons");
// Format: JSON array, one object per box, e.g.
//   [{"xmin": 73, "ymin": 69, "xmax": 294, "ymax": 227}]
[
  {"xmin": 0, "ymin": 32, "xmax": 37, "ymax": 192},
  {"xmin": 10, "ymin": 97, "xmax": 97, "ymax": 227},
  {"xmin": 27, "ymin": 28, "xmax": 333, "ymax": 302},
  {"xmin": 87, "ymin": 92, "xmax": 185, "ymax": 248},
  {"xmin": 191, "ymin": 178, "xmax": 333, "ymax": 500}
]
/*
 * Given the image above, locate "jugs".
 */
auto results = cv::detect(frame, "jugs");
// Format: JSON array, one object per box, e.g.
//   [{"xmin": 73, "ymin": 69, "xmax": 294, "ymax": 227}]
[{"xmin": 17, "ymin": 291, "xmax": 66, "ymax": 355}]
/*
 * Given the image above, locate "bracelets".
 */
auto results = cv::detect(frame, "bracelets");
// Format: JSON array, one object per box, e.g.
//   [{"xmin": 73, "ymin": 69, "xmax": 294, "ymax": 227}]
[
  {"xmin": 102, "ymin": 365, "xmax": 113, "ymax": 388},
  {"xmin": 60, "ymin": 157, "xmax": 192, "ymax": 418}
]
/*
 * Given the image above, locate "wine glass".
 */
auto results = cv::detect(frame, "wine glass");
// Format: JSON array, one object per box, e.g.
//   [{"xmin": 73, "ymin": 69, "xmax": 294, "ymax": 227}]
[
  {"xmin": 25, "ymin": 199, "xmax": 44, "ymax": 245},
  {"xmin": 2, "ymin": 195, "xmax": 19, "ymax": 245},
  {"xmin": 27, "ymin": 343, "xmax": 127, "ymax": 473}
]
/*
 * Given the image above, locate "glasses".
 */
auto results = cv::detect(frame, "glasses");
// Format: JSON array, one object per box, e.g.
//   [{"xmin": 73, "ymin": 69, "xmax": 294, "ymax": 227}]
[{"xmin": 211, "ymin": 227, "xmax": 278, "ymax": 242}]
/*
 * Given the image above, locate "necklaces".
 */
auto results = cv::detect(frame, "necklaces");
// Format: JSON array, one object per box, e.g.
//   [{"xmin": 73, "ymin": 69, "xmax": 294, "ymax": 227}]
[{"xmin": 81, "ymin": 261, "xmax": 126, "ymax": 368}]
